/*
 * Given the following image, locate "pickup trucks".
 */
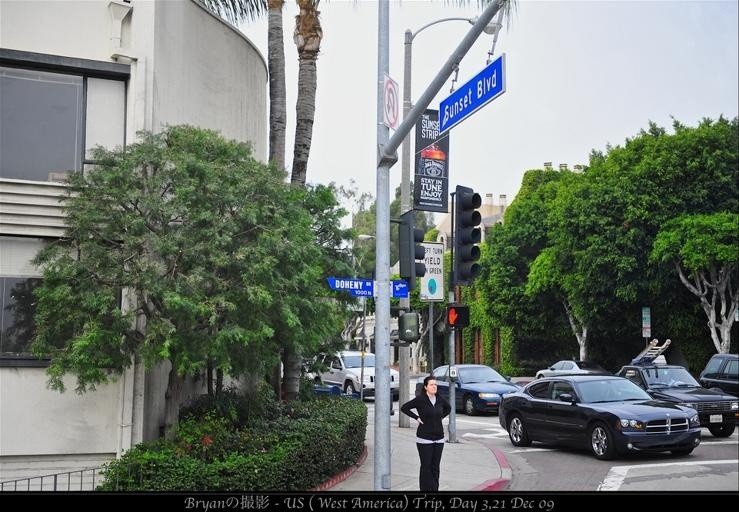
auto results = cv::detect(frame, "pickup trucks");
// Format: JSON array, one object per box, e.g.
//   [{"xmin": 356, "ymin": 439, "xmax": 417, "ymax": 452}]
[{"xmin": 614, "ymin": 365, "xmax": 739, "ymax": 438}]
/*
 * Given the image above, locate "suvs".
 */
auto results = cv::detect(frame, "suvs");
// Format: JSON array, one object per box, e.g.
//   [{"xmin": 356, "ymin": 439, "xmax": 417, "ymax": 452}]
[
  {"xmin": 700, "ymin": 353, "xmax": 739, "ymax": 395},
  {"xmin": 309, "ymin": 350, "xmax": 399, "ymax": 401}
]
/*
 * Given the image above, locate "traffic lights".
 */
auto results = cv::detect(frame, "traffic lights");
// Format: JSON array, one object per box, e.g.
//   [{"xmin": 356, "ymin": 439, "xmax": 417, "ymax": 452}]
[
  {"xmin": 390, "ymin": 211, "xmax": 425, "ymax": 291},
  {"xmin": 453, "ymin": 185, "xmax": 482, "ymax": 285},
  {"xmin": 445, "ymin": 305, "xmax": 470, "ymax": 328}
]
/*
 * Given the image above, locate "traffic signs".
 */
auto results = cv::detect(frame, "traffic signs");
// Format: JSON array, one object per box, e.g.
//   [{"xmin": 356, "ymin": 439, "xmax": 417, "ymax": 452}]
[
  {"xmin": 438, "ymin": 53, "xmax": 506, "ymax": 136},
  {"xmin": 418, "ymin": 241, "xmax": 445, "ymax": 302},
  {"xmin": 327, "ymin": 278, "xmax": 408, "ymax": 298}
]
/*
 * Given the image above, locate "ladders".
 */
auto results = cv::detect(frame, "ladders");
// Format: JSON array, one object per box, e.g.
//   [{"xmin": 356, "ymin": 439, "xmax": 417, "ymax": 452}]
[{"xmin": 614, "ymin": 339, "xmax": 671, "ymax": 379}]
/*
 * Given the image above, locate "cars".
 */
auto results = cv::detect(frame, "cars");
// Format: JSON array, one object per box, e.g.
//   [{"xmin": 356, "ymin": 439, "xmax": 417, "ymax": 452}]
[
  {"xmin": 415, "ymin": 364, "xmax": 521, "ymax": 415},
  {"xmin": 535, "ymin": 360, "xmax": 612, "ymax": 379},
  {"xmin": 499, "ymin": 376, "xmax": 700, "ymax": 459}
]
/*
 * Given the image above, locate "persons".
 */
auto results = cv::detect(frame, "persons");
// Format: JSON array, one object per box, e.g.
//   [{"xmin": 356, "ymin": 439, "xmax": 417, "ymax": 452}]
[{"xmin": 401, "ymin": 376, "xmax": 452, "ymax": 492}]
